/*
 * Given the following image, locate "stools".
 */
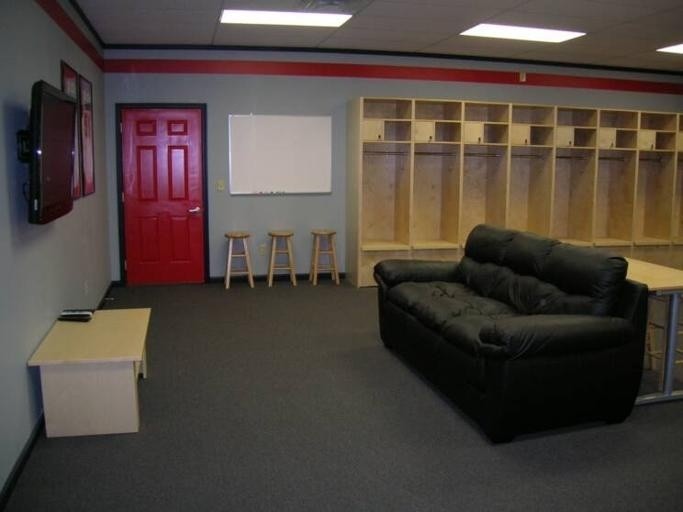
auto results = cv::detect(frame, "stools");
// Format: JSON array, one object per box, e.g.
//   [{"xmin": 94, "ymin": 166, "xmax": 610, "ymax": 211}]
[{"xmin": 224, "ymin": 229, "xmax": 341, "ymax": 291}]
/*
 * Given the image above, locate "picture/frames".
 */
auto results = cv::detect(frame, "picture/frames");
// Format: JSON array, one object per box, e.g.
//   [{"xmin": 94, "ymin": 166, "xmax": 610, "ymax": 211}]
[{"xmin": 58, "ymin": 57, "xmax": 96, "ymax": 201}]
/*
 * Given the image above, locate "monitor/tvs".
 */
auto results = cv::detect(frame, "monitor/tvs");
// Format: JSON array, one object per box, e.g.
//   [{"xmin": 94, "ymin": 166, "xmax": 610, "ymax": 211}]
[{"xmin": 17, "ymin": 79, "xmax": 78, "ymax": 225}]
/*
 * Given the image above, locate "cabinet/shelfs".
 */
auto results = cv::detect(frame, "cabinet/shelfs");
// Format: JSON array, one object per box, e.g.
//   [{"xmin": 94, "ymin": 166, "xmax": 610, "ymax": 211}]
[{"xmin": 344, "ymin": 95, "xmax": 683, "ymax": 291}]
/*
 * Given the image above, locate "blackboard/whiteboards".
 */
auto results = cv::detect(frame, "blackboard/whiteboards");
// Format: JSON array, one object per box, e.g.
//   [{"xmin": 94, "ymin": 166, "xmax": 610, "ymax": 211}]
[{"xmin": 228, "ymin": 114, "xmax": 333, "ymax": 195}]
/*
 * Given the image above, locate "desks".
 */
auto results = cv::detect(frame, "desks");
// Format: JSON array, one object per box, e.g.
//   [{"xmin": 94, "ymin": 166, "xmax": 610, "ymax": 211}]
[
  {"xmin": 620, "ymin": 254, "xmax": 683, "ymax": 405},
  {"xmin": 29, "ymin": 308, "xmax": 153, "ymax": 439}
]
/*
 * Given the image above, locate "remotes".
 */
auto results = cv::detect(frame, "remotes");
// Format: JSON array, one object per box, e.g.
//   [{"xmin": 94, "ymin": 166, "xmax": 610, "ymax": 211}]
[{"xmin": 58, "ymin": 308, "xmax": 95, "ymax": 322}]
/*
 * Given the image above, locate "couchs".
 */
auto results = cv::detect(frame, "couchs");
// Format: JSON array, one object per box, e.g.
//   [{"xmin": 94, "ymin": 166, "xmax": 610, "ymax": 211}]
[{"xmin": 371, "ymin": 224, "xmax": 648, "ymax": 445}]
